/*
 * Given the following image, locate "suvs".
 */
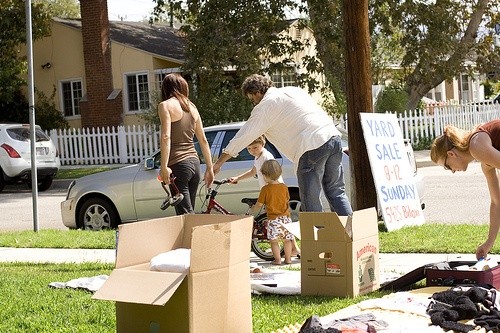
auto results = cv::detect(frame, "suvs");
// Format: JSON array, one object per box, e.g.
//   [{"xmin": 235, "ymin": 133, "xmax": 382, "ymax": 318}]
[{"xmin": 0, "ymin": 122, "xmax": 61, "ymax": 191}]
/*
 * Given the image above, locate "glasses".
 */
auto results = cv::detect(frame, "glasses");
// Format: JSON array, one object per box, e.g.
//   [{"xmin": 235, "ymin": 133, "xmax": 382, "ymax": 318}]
[
  {"xmin": 249, "ymin": 96, "xmax": 256, "ymax": 103},
  {"xmin": 444, "ymin": 146, "xmax": 454, "ymax": 170}
]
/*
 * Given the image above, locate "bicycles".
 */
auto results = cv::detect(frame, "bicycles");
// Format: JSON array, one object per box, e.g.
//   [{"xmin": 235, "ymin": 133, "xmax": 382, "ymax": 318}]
[{"xmin": 200, "ymin": 179, "xmax": 286, "ymax": 261}]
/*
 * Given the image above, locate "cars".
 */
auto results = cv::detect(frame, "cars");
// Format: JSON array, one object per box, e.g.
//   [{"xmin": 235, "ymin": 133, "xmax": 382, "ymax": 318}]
[{"xmin": 60, "ymin": 120, "xmax": 351, "ymax": 230}]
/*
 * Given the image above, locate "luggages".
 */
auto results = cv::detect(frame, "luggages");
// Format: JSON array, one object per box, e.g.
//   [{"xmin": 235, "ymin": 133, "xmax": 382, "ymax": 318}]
[{"xmin": 379, "ymin": 261, "xmax": 500, "ymax": 289}]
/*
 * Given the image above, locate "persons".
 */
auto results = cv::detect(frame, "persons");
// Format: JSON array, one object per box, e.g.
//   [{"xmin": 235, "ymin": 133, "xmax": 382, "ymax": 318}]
[
  {"xmin": 158, "ymin": 73, "xmax": 214, "ymax": 214},
  {"xmin": 250, "ymin": 160, "xmax": 294, "ymax": 265},
  {"xmin": 214, "ymin": 74, "xmax": 352, "ymax": 229},
  {"xmin": 430, "ymin": 120, "xmax": 500, "ymax": 260},
  {"xmin": 231, "ymin": 133, "xmax": 301, "ymax": 257}
]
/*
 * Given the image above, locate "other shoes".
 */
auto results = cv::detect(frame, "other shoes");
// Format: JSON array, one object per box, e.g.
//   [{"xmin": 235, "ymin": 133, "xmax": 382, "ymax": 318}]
[
  {"xmin": 264, "ymin": 246, "xmax": 281, "ymax": 253},
  {"xmin": 282, "ymin": 249, "xmax": 300, "ymax": 258},
  {"xmin": 271, "ymin": 260, "xmax": 281, "ymax": 264},
  {"xmin": 284, "ymin": 260, "xmax": 291, "ymax": 263}
]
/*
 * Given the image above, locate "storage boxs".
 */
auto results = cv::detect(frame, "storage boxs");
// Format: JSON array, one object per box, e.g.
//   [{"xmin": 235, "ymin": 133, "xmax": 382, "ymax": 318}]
[
  {"xmin": 299, "ymin": 207, "xmax": 380, "ymax": 299},
  {"xmin": 90, "ymin": 213, "xmax": 254, "ymax": 333}
]
